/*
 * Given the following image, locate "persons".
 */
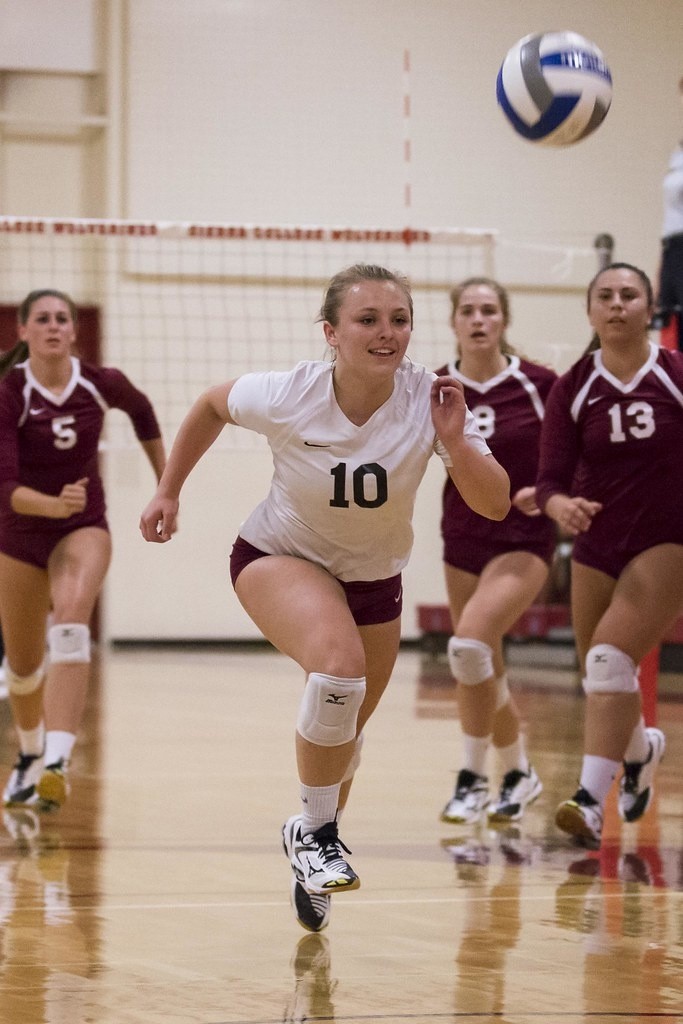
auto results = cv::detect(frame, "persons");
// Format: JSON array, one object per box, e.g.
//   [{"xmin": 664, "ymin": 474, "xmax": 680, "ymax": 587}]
[
  {"xmin": 0, "ymin": 290, "xmax": 167, "ymax": 813},
  {"xmin": 433, "ymin": 276, "xmax": 557, "ymax": 828},
  {"xmin": 535, "ymin": 261, "xmax": 683, "ymax": 851},
  {"xmin": 140, "ymin": 263, "xmax": 510, "ymax": 934}
]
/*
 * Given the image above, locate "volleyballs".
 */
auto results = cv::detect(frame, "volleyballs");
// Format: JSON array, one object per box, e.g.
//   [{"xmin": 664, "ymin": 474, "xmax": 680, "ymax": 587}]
[{"xmin": 496, "ymin": 28, "xmax": 615, "ymax": 147}]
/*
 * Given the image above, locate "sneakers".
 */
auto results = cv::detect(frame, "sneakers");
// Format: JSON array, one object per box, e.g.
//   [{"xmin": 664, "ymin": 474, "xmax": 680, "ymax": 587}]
[
  {"xmin": 487, "ymin": 764, "xmax": 543, "ymax": 821},
  {"xmin": 617, "ymin": 727, "xmax": 665, "ymax": 822},
  {"xmin": 555, "ymin": 778, "xmax": 605, "ymax": 850},
  {"xmin": 442, "ymin": 769, "xmax": 491, "ymax": 825},
  {"xmin": 35, "ymin": 759, "xmax": 72, "ymax": 813},
  {"xmin": 282, "ymin": 814, "xmax": 331, "ymax": 932},
  {"xmin": 3, "ymin": 751, "xmax": 44, "ymax": 804},
  {"xmin": 291, "ymin": 808, "xmax": 360, "ymax": 894}
]
